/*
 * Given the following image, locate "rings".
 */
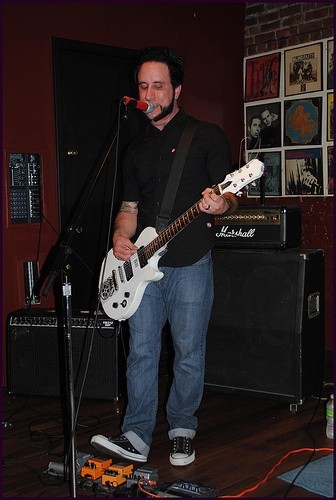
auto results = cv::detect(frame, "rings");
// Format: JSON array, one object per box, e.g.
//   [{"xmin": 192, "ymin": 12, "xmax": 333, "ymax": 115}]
[{"xmin": 206, "ymin": 205, "xmax": 210, "ymax": 210}]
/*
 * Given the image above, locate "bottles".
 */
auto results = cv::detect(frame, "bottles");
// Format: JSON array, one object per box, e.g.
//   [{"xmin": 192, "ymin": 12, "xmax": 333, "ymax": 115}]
[{"xmin": 326, "ymin": 394, "xmax": 334, "ymax": 439}]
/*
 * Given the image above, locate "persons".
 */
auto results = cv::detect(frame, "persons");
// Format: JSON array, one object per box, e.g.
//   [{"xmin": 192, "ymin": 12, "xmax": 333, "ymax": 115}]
[
  {"xmin": 248, "ymin": 105, "xmax": 280, "ymax": 148},
  {"xmin": 89, "ymin": 47, "xmax": 239, "ymax": 466}
]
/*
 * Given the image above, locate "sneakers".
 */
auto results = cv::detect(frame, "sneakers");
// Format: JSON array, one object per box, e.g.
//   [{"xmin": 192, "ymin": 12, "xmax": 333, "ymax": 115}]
[
  {"xmin": 91, "ymin": 433, "xmax": 148, "ymax": 463},
  {"xmin": 169, "ymin": 436, "xmax": 195, "ymax": 466}
]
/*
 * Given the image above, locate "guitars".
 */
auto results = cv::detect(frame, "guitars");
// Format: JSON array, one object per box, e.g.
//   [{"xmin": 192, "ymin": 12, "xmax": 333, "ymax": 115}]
[{"xmin": 98, "ymin": 158, "xmax": 265, "ymax": 322}]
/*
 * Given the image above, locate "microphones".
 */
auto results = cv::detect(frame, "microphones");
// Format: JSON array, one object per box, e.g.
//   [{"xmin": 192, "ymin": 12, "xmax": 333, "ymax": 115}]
[{"xmin": 123, "ymin": 95, "xmax": 154, "ymax": 114}]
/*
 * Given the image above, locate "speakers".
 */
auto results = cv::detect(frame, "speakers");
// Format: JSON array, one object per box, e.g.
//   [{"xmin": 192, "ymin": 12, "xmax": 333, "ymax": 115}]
[
  {"xmin": 203, "ymin": 247, "xmax": 326, "ymax": 416},
  {"xmin": 6, "ymin": 308, "xmax": 129, "ymax": 403}
]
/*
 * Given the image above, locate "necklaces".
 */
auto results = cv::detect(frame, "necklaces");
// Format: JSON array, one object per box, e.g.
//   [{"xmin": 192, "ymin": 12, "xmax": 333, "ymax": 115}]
[{"xmin": 154, "ymin": 123, "xmax": 167, "ymax": 126}]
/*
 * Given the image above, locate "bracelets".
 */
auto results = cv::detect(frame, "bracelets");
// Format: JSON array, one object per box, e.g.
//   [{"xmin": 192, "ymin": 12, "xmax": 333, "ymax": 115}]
[{"xmin": 222, "ymin": 199, "xmax": 233, "ymax": 215}]
